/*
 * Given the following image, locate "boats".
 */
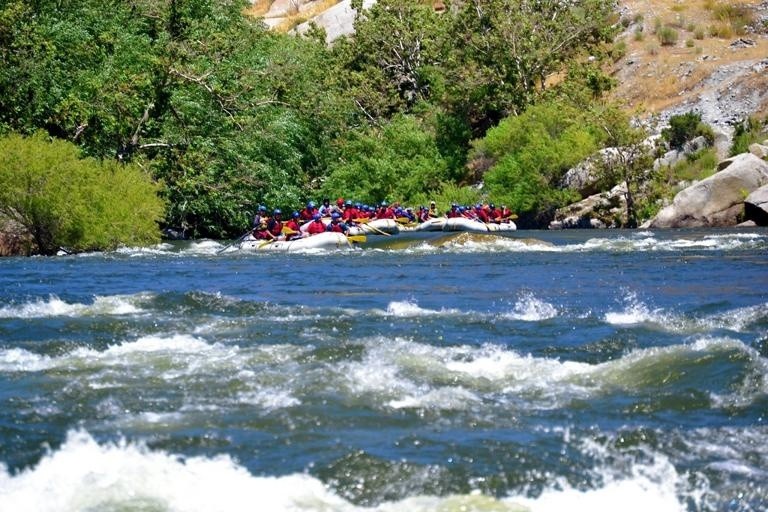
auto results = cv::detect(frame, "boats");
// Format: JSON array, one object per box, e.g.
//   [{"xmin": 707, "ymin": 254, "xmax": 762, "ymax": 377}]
[
  {"xmin": 400, "ymin": 218, "xmax": 518, "ymax": 232},
  {"xmin": 299, "ymin": 216, "xmax": 399, "ymax": 235},
  {"xmin": 240, "ymin": 232, "xmax": 351, "ymax": 254}
]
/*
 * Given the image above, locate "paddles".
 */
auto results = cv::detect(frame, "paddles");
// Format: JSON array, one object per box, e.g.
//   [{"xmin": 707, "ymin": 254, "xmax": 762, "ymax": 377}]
[
  {"xmin": 365, "ymin": 224, "xmax": 391, "ymax": 237},
  {"xmin": 259, "ymin": 238, "xmax": 274, "ymax": 249},
  {"xmin": 466, "ymin": 211, "xmax": 493, "ymax": 230},
  {"xmin": 352, "ymin": 218, "xmax": 368, "ymax": 223},
  {"xmin": 348, "ymin": 236, "xmax": 366, "ymax": 242},
  {"xmin": 392, "ymin": 217, "xmax": 409, "ymax": 222},
  {"xmin": 281, "ymin": 227, "xmax": 301, "ymax": 234},
  {"xmin": 402, "ymin": 222, "xmax": 417, "ymax": 226},
  {"xmin": 216, "ymin": 224, "xmax": 262, "ymax": 252},
  {"xmin": 501, "ymin": 214, "xmax": 518, "ymax": 220}
]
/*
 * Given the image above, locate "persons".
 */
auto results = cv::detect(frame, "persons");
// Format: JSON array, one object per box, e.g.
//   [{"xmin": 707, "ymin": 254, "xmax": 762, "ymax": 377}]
[{"xmin": 252, "ymin": 196, "xmax": 515, "ymax": 242}]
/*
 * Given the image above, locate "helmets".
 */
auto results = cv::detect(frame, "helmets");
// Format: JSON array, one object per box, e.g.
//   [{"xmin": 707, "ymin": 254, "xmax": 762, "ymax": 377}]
[
  {"xmin": 337, "ymin": 198, "xmax": 411, "ymax": 213},
  {"xmin": 293, "ymin": 211, "xmax": 299, "ymax": 218},
  {"xmin": 323, "ymin": 198, "xmax": 329, "ymax": 205},
  {"xmin": 452, "ymin": 203, "xmax": 505, "ymax": 212},
  {"xmin": 332, "ymin": 213, "xmax": 340, "ymax": 222},
  {"xmin": 257, "ymin": 206, "xmax": 266, "ymax": 211},
  {"xmin": 420, "ymin": 201, "xmax": 435, "ymax": 211},
  {"xmin": 307, "ymin": 202, "xmax": 316, "ymax": 209},
  {"xmin": 313, "ymin": 214, "xmax": 321, "ymax": 220},
  {"xmin": 274, "ymin": 209, "xmax": 282, "ymax": 216}
]
